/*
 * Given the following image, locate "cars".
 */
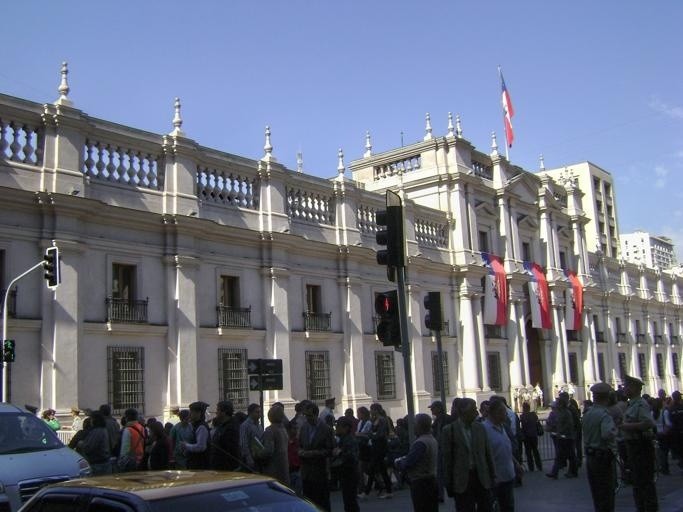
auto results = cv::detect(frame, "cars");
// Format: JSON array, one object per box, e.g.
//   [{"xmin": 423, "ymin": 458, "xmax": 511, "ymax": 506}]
[{"xmin": 14, "ymin": 466, "xmax": 325, "ymax": 512}]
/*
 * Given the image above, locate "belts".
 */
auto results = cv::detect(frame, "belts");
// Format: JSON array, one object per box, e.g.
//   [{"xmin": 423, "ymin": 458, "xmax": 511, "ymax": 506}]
[
  {"xmin": 94, "ymin": 457, "xmax": 111, "ymax": 464},
  {"xmin": 628, "ymin": 439, "xmax": 656, "ymax": 446},
  {"xmin": 583, "ymin": 447, "xmax": 614, "ymax": 457}
]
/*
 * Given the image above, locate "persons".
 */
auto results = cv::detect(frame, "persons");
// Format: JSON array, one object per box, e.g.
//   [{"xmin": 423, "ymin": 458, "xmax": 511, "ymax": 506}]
[
  {"xmin": 239, "ymin": 394, "xmax": 543, "ymax": 512},
  {"xmin": 25, "ymin": 400, "xmax": 248, "ymax": 472},
  {"xmin": 545, "ymin": 373, "xmax": 683, "ymax": 512}
]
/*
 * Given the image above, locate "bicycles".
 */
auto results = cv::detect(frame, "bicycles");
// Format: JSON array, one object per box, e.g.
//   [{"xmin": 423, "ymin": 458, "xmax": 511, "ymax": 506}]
[{"xmin": 613, "ymin": 444, "xmax": 661, "ymax": 497}]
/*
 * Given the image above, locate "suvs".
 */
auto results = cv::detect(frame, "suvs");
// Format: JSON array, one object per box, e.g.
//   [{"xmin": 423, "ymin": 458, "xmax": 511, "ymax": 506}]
[{"xmin": 0, "ymin": 398, "xmax": 93, "ymax": 512}]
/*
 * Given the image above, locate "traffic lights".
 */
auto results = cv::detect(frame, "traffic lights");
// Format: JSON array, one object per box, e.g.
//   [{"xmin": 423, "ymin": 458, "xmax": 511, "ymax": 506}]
[
  {"xmin": 3, "ymin": 338, "xmax": 16, "ymax": 363},
  {"xmin": 373, "ymin": 205, "xmax": 405, "ymax": 270},
  {"xmin": 422, "ymin": 292, "xmax": 443, "ymax": 333},
  {"xmin": 373, "ymin": 289, "xmax": 401, "ymax": 350},
  {"xmin": 43, "ymin": 244, "xmax": 60, "ymax": 291}
]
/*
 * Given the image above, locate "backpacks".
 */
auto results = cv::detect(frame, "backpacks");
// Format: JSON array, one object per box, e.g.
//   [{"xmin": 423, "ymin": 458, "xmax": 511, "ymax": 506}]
[
  {"xmin": 117, "ymin": 454, "xmax": 148, "ymax": 472},
  {"xmin": 147, "ymin": 447, "xmax": 166, "ymax": 470}
]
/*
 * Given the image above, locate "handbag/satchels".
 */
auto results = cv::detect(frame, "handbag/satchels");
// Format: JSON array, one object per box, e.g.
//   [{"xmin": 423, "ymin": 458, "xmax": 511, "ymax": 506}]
[
  {"xmin": 81, "ymin": 449, "xmax": 96, "ymax": 472},
  {"xmin": 187, "ymin": 442, "xmax": 217, "ymax": 469},
  {"xmin": 445, "ymin": 472, "xmax": 456, "ymax": 498},
  {"xmin": 536, "ymin": 422, "xmax": 544, "ymax": 436},
  {"xmin": 351, "ymin": 461, "xmax": 384, "ymax": 488}
]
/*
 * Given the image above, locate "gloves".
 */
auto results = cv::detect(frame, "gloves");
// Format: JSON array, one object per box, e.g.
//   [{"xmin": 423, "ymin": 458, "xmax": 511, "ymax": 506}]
[{"xmin": 623, "ymin": 372, "xmax": 646, "ymax": 385}]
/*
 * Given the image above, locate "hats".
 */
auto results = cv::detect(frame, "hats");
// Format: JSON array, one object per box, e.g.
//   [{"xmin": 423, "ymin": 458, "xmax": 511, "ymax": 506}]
[
  {"xmin": 335, "ymin": 417, "xmax": 352, "ymax": 426},
  {"xmin": 71, "ymin": 406, "xmax": 80, "ymax": 413},
  {"xmin": 325, "ymin": 397, "xmax": 336, "ymax": 402},
  {"xmin": 591, "ymin": 383, "xmax": 612, "ymax": 394},
  {"xmin": 189, "ymin": 401, "xmax": 210, "ymax": 409},
  {"xmin": 672, "ymin": 390, "xmax": 683, "ymax": 397},
  {"xmin": 428, "ymin": 400, "xmax": 443, "ymax": 408},
  {"xmin": 25, "ymin": 403, "xmax": 38, "ymax": 411}
]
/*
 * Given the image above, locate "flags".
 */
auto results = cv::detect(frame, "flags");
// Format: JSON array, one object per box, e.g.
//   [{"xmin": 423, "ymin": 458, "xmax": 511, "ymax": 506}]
[{"xmin": 499, "ymin": 73, "xmax": 515, "ymax": 148}]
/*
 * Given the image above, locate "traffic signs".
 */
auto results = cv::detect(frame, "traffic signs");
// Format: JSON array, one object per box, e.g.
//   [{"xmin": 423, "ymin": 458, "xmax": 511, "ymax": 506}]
[
  {"xmin": 247, "ymin": 358, "xmax": 284, "ymax": 375},
  {"xmin": 248, "ymin": 374, "xmax": 283, "ymax": 393}
]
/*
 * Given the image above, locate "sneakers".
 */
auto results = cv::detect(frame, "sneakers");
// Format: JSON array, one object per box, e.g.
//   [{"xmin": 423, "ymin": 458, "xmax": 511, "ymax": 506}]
[
  {"xmin": 528, "ymin": 465, "xmax": 542, "ymax": 472},
  {"xmin": 513, "ymin": 478, "xmax": 522, "ymax": 488},
  {"xmin": 659, "ymin": 463, "xmax": 683, "ymax": 475}
]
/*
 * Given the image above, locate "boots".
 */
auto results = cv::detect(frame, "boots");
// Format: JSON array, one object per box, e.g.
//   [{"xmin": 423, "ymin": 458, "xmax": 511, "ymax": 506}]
[
  {"xmin": 564, "ymin": 464, "xmax": 578, "ymax": 478},
  {"xmin": 545, "ymin": 465, "xmax": 561, "ymax": 479}
]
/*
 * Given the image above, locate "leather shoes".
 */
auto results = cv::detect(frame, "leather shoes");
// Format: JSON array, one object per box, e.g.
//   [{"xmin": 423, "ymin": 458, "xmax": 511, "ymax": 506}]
[
  {"xmin": 376, "ymin": 493, "xmax": 393, "ymax": 499},
  {"xmin": 357, "ymin": 493, "xmax": 369, "ymax": 501}
]
